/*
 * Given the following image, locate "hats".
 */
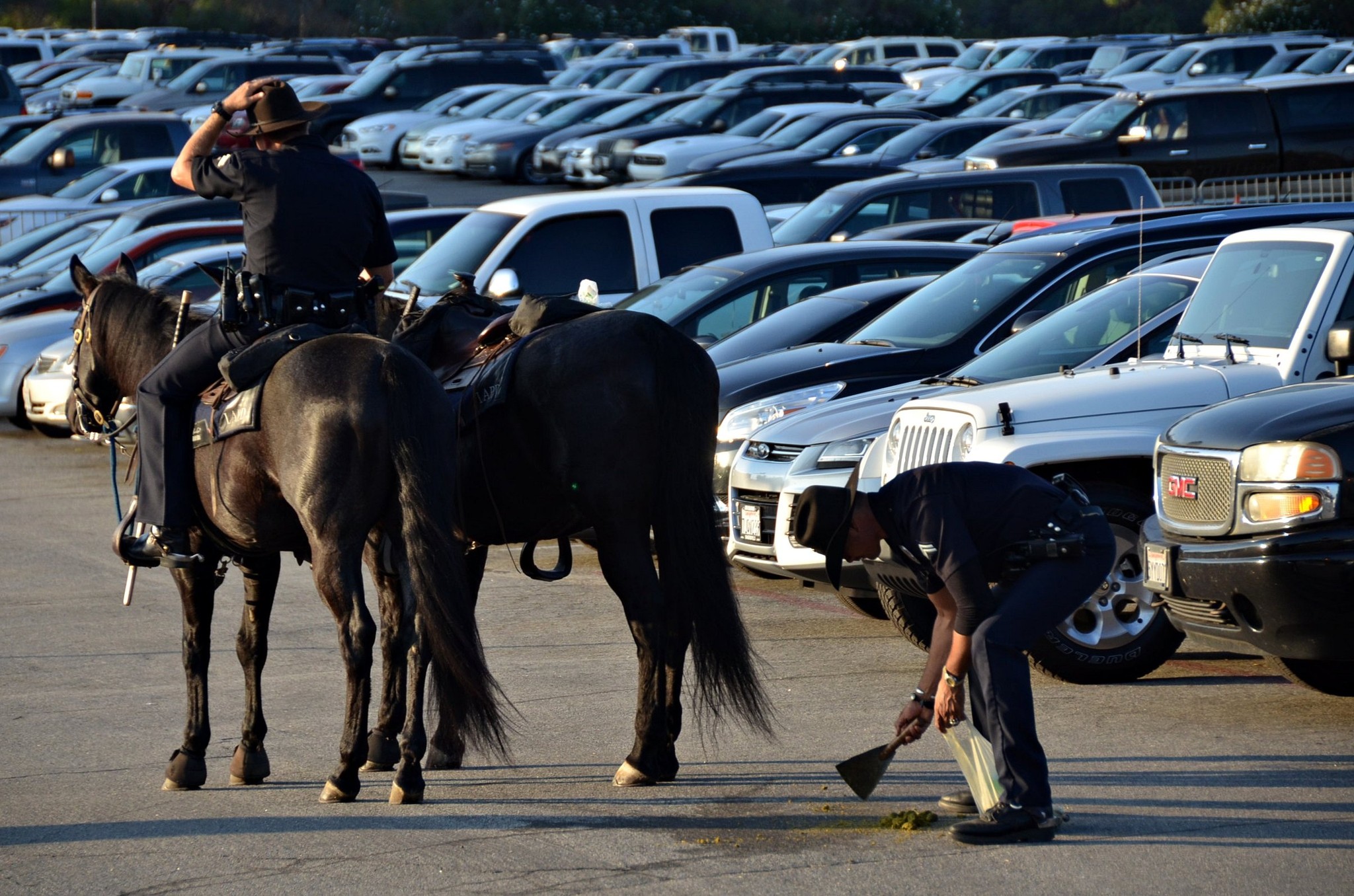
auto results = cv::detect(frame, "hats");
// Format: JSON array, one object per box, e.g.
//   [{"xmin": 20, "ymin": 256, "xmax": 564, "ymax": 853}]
[
  {"xmin": 226, "ymin": 79, "xmax": 330, "ymax": 137},
  {"xmin": 794, "ymin": 460, "xmax": 861, "ymax": 592}
]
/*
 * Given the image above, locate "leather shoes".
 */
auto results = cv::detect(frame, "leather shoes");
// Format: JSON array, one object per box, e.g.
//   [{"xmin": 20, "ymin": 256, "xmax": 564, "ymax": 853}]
[{"xmin": 119, "ymin": 525, "xmax": 186, "ymax": 567}]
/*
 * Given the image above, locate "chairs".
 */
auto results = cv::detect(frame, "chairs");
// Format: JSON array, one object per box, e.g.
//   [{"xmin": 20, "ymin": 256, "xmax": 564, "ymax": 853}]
[
  {"xmin": 1100, "ymin": 286, "xmax": 1179, "ymax": 346},
  {"xmin": 98, "ymin": 134, "xmax": 125, "ymax": 166}
]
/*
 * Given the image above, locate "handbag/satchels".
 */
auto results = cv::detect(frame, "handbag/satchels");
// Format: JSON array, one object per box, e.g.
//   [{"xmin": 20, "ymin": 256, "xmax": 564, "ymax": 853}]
[{"xmin": 941, "ymin": 713, "xmax": 1005, "ymax": 818}]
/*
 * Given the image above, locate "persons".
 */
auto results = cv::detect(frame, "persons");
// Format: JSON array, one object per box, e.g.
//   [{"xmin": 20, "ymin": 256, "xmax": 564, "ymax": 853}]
[
  {"xmin": 792, "ymin": 462, "xmax": 1116, "ymax": 847},
  {"xmin": 122, "ymin": 78, "xmax": 401, "ymax": 569}
]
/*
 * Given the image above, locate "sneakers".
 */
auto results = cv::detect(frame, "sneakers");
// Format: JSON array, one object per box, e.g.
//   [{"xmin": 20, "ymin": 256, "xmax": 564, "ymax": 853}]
[
  {"xmin": 948, "ymin": 802, "xmax": 1054, "ymax": 845},
  {"xmin": 938, "ymin": 789, "xmax": 979, "ymax": 814}
]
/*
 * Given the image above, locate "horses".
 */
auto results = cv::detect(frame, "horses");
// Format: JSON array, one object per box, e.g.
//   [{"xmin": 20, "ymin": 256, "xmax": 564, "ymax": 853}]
[
  {"xmin": 193, "ymin": 251, "xmax": 788, "ymax": 788},
  {"xmin": 64, "ymin": 252, "xmax": 528, "ymax": 806}
]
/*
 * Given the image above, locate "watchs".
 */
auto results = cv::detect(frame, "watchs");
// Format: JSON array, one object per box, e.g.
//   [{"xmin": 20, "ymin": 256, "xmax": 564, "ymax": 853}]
[
  {"xmin": 209, "ymin": 98, "xmax": 231, "ymax": 122},
  {"xmin": 940, "ymin": 665, "xmax": 963, "ymax": 689}
]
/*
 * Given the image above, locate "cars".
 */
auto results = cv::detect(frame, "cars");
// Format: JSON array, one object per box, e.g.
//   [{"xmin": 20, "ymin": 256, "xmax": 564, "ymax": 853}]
[{"xmin": 1, "ymin": 15, "xmax": 1354, "ymax": 696}]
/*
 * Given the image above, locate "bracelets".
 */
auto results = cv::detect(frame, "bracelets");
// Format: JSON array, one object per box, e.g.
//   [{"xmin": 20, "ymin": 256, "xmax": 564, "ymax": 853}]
[{"xmin": 910, "ymin": 686, "xmax": 935, "ymax": 711}]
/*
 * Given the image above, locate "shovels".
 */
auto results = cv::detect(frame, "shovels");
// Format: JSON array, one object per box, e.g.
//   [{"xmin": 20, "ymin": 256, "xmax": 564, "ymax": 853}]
[{"xmin": 835, "ymin": 719, "xmax": 921, "ymax": 802}]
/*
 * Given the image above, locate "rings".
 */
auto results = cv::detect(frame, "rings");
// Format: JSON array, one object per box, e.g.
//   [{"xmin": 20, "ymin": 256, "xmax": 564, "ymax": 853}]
[{"xmin": 949, "ymin": 718, "xmax": 959, "ymax": 725}]
